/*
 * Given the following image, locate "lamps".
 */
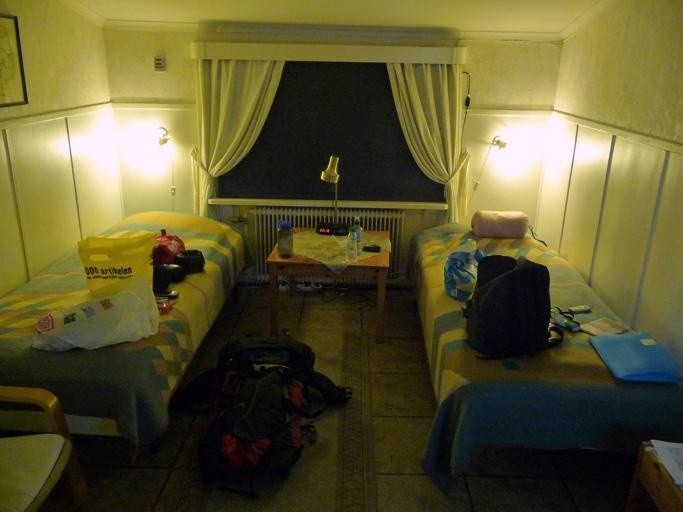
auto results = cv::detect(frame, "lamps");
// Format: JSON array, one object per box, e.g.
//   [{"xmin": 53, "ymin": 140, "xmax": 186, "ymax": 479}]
[
  {"xmin": 472, "ymin": 135, "xmax": 509, "ymax": 194},
  {"xmin": 320, "ymin": 154, "xmax": 341, "ymax": 222},
  {"xmin": 147, "ymin": 127, "xmax": 176, "ymax": 195}
]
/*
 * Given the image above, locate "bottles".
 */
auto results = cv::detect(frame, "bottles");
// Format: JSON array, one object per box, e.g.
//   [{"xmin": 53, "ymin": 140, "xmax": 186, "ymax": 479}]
[
  {"xmin": 276, "ymin": 220, "xmax": 293, "ymax": 259},
  {"xmin": 347, "ymin": 216, "xmax": 362, "ymax": 258}
]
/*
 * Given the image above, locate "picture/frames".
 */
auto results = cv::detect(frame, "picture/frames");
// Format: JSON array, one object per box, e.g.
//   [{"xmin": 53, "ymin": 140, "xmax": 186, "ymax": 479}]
[{"xmin": 0, "ymin": 15, "xmax": 29, "ymax": 110}]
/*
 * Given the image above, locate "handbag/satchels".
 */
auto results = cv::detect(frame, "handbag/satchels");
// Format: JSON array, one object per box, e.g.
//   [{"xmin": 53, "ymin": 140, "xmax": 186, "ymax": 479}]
[
  {"xmin": 444, "ymin": 238, "xmax": 483, "ymax": 303},
  {"xmin": 200, "ymin": 334, "xmax": 352, "ymax": 500},
  {"xmin": 152, "ymin": 230, "xmax": 185, "ymax": 264}
]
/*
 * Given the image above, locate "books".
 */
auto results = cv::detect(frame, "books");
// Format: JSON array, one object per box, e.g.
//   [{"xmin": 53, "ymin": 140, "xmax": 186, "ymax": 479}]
[{"xmin": 578, "ymin": 316, "xmax": 630, "ymax": 340}]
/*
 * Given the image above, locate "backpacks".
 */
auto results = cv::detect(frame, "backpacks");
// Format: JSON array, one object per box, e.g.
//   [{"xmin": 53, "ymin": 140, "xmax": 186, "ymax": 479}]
[{"xmin": 464, "ymin": 254, "xmax": 564, "ymax": 362}]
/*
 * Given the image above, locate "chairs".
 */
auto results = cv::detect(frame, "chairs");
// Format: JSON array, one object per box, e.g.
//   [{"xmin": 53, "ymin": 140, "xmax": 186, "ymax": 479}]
[{"xmin": 0, "ymin": 382, "xmax": 89, "ymax": 511}]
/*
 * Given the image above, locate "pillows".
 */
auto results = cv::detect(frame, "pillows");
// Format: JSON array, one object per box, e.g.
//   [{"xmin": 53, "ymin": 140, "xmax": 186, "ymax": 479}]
[{"xmin": 473, "ymin": 211, "xmax": 533, "ymax": 237}]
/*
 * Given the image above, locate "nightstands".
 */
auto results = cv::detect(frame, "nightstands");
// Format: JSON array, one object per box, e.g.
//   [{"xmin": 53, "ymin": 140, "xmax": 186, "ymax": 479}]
[{"xmin": 625, "ymin": 439, "xmax": 683, "ymax": 511}]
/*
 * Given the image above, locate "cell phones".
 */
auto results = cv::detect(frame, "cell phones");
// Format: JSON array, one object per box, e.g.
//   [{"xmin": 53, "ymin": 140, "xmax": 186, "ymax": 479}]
[
  {"xmin": 363, "ymin": 246, "xmax": 380, "ymax": 252},
  {"xmin": 568, "ymin": 305, "xmax": 591, "ymax": 314},
  {"xmin": 154, "ymin": 290, "xmax": 178, "ymax": 299}
]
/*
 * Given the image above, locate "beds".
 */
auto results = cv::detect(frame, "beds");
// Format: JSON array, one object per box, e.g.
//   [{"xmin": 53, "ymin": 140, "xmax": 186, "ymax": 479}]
[
  {"xmin": 0, "ymin": 211, "xmax": 243, "ymax": 440},
  {"xmin": 411, "ymin": 222, "xmax": 683, "ymax": 483}
]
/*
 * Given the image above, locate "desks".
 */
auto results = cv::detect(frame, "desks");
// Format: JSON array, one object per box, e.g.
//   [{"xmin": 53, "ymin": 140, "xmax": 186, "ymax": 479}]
[{"xmin": 266, "ymin": 227, "xmax": 391, "ymax": 334}]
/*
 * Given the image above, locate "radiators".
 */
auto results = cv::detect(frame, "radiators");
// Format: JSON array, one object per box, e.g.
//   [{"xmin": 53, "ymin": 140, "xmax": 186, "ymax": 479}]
[{"xmin": 243, "ymin": 208, "xmax": 406, "ymax": 286}]
